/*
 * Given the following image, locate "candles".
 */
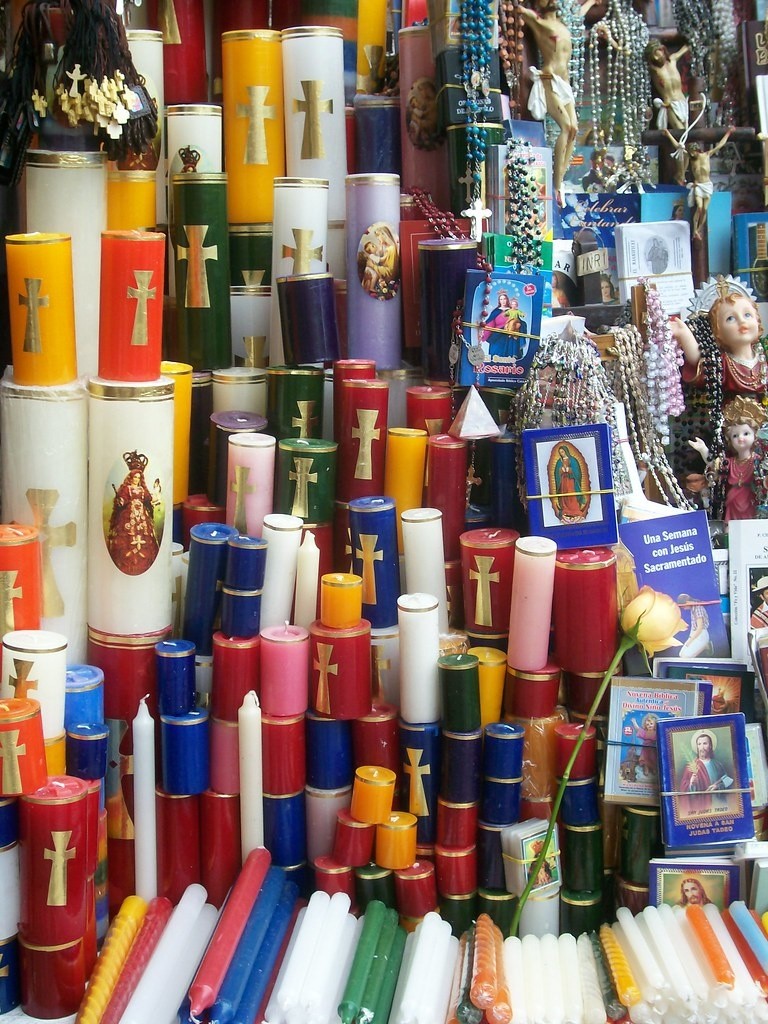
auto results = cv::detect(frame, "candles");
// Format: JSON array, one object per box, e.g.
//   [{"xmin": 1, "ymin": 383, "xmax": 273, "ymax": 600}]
[{"xmin": 0, "ymin": 0, "xmax": 768, "ymax": 1023}]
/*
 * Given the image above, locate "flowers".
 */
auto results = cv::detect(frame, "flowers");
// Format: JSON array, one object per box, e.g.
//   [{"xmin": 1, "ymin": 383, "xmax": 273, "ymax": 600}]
[{"xmin": 507, "ymin": 584, "xmax": 688, "ymax": 936}]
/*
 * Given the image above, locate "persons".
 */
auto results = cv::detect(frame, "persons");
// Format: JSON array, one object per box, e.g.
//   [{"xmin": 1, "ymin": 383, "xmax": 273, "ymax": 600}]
[
  {"xmin": 751, "ymin": 576, "xmax": 768, "ymax": 630},
  {"xmin": 678, "ymin": 593, "xmax": 714, "ymax": 660},
  {"xmin": 519, "ymin": 0, "xmax": 598, "ymax": 209},
  {"xmin": 667, "ymin": 193, "xmax": 686, "ymax": 220},
  {"xmin": 668, "ymin": 273, "xmax": 768, "ymax": 530},
  {"xmin": 581, "ymin": 148, "xmax": 617, "ymax": 195},
  {"xmin": 599, "ymin": 24, "xmax": 690, "ymax": 188},
  {"xmin": 662, "ymin": 127, "xmax": 741, "ymax": 242},
  {"xmin": 552, "ymin": 273, "xmax": 616, "ymax": 308}
]
